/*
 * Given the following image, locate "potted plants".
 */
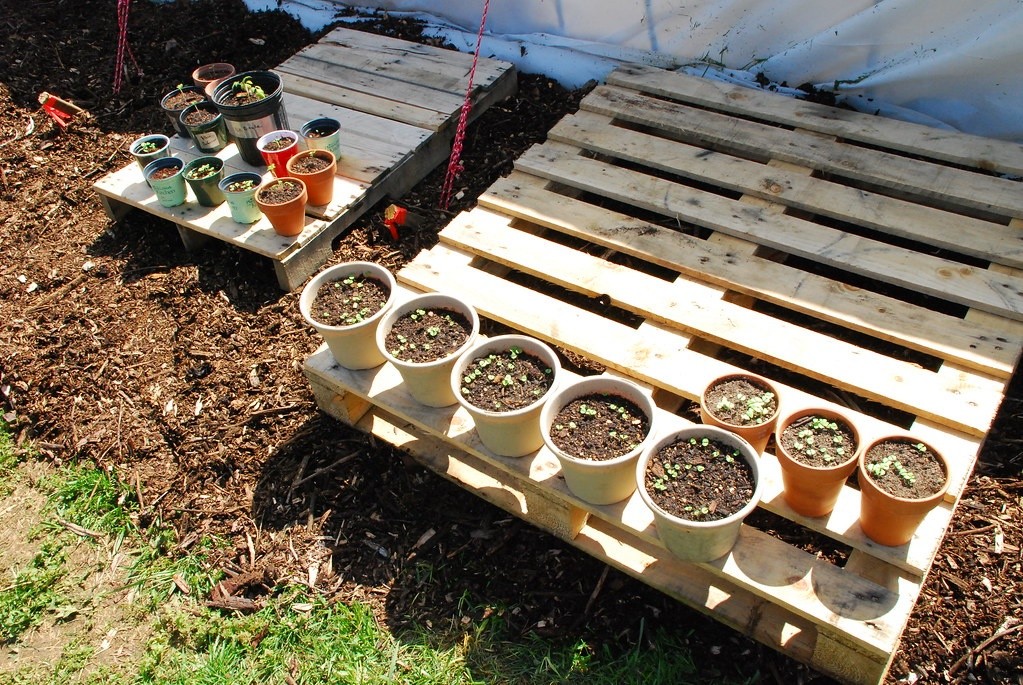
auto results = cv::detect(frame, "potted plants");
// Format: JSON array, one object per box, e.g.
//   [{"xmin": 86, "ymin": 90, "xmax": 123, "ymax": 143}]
[
  {"xmin": 451, "ymin": 333, "xmax": 560, "ymax": 458},
  {"xmin": 256, "ymin": 130, "xmax": 299, "ymax": 179},
  {"xmin": 218, "ymin": 171, "xmax": 264, "ymax": 224},
  {"xmin": 211, "ymin": 69, "xmax": 291, "ymax": 167},
  {"xmin": 286, "ymin": 149, "xmax": 337, "ymax": 207},
  {"xmin": 700, "ymin": 373, "xmax": 780, "ymax": 458},
  {"xmin": 299, "ymin": 117, "xmax": 341, "ymax": 162},
  {"xmin": 375, "ymin": 291, "xmax": 480, "ymax": 408},
  {"xmin": 192, "ymin": 63, "xmax": 236, "ymax": 96},
  {"xmin": 300, "ymin": 261, "xmax": 398, "ymax": 371},
  {"xmin": 182, "ymin": 156, "xmax": 224, "ymax": 207},
  {"xmin": 143, "ymin": 157, "xmax": 188, "ymax": 208},
  {"xmin": 857, "ymin": 433, "xmax": 950, "ymax": 547},
  {"xmin": 179, "ymin": 100, "xmax": 227, "ymax": 154},
  {"xmin": 776, "ymin": 407, "xmax": 864, "ymax": 518},
  {"xmin": 255, "ymin": 163, "xmax": 310, "ymax": 237},
  {"xmin": 635, "ymin": 423, "xmax": 764, "ymax": 564},
  {"xmin": 161, "ymin": 84, "xmax": 208, "ymax": 140},
  {"xmin": 130, "ymin": 133, "xmax": 172, "ymax": 188},
  {"xmin": 539, "ymin": 375, "xmax": 657, "ymax": 505}
]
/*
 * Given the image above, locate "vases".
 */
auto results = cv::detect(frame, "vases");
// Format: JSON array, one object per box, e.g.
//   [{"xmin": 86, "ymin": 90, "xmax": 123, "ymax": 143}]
[{"xmin": 205, "ymin": 76, "xmax": 230, "ymax": 96}]
